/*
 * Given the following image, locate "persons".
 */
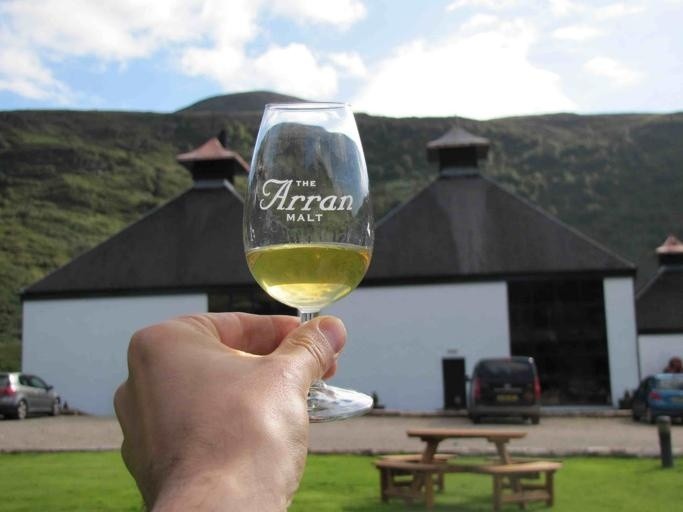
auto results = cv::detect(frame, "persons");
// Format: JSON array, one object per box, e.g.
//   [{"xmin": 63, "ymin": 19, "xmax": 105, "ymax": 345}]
[{"xmin": 112, "ymin": 311, "xmax": 347, "ymax": 512}]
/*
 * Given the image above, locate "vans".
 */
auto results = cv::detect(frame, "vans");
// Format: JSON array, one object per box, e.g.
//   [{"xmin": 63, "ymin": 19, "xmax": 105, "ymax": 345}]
[{"xmin": 464, "ymin": 354, "xmax": 543, "ymax": 425}]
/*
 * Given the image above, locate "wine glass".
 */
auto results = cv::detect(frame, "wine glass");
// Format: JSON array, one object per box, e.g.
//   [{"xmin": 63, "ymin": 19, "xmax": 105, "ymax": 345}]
[{"xmin": 240, "ymin": 98, "xmax": 376, "ymax": 424}]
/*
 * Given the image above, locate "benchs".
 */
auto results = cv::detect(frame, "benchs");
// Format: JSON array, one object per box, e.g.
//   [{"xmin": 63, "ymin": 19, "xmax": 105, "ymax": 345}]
[{"xmin": 374, "ymin": 428, "xmax": 562, "ymax": 511}]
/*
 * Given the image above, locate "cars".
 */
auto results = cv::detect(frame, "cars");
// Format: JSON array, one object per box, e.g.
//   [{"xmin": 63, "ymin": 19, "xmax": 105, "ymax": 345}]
[
  {"xmin": 632, "ymin": 371, "xmax": 683, "ymax": 424},
  {"xmin": 0, "ymin": 369, "xmax": 61, "ymax": 419}
]
